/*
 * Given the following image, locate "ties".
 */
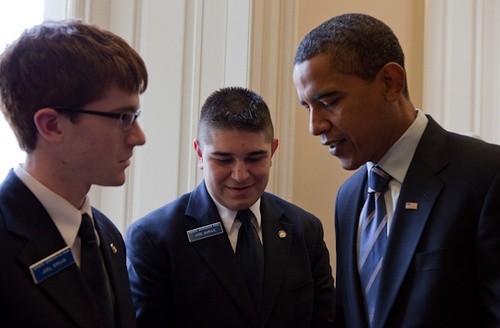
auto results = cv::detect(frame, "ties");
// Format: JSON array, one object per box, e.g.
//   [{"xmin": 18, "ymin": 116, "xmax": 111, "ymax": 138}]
[
  {"xmin": 235, "ymin": 208, "xmax": 262, "ymax": 328},
  {"xmin": 357, "ymin": 166, "xmax": 395, "ymax": 328},
  {"xmin": 77, "ymin": 213, "xmax": 116, "ymax": 328}
]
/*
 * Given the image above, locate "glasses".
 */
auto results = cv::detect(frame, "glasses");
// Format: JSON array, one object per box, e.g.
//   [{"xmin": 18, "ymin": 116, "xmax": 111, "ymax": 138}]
[{"xmin": 52, "ymin": 107, "xmax": 141, "ymax": 131}]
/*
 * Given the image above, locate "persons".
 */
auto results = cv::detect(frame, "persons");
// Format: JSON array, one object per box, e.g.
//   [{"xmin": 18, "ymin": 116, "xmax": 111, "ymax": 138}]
[
  {"xmin": 0, "ymin": 19, "xmax": 148, "ymax": 328},
  {"xmin": 293, "ymin": 12, "xmax": 500, "ymax": 328},
  {"xmin": 126, "ymin": 87, "xmax": 339, "ymax": 328}
]
